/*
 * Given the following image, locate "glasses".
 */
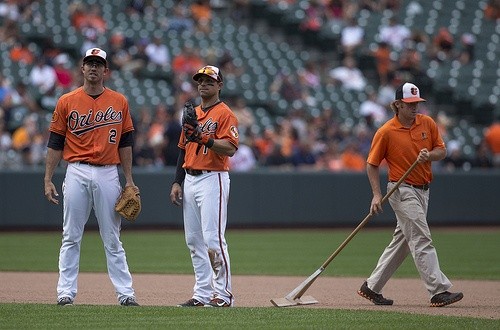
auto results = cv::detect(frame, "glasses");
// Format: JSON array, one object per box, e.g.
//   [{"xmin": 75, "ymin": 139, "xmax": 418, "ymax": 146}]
[{"xmin": 195, "ymin": 67, "xmax": 222, "ymax": 82}]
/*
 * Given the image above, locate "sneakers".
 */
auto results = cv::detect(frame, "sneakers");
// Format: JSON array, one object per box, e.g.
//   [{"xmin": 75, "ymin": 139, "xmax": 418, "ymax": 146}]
[
  {"xmin": 176, "ymin": 298, "xmax": 204, "ymax": 308},
  {"xmin": 120, "ymin": 296, "xmax": 141, "ymax": 307},
  {"xmin": 57, "ymin": 296, "xmax": 74, "ymax": 306},
  {"xmin": 429, "ymin": 290, "xmax": 465, "ymax": 307},
  {"xmin": 203, "ymin": 298, "xmax": 229, "ymax": 308},
  {"xmin": 356, "ymin": 280, "xmax": 395, "ymax": 306}
]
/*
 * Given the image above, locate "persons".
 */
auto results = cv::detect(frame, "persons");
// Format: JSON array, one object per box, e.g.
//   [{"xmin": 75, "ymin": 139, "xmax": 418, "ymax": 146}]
[
  {"xmin": 170, "ymin": 65, "xmax": 239, "ymax": 308},
  {"xmin": 45, "ymin": 47, "xmax": 141, "ymax": 307},
  {"xmin": 0, "ymin": 0, "xmax": 500, "ymax": 169},
  {"xmin": 356, "ymin": 82, "xmax": 464, "ymax": 307}
]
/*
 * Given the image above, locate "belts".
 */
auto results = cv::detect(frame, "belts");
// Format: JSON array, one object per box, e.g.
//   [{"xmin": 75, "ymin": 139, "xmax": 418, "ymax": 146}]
[
  {"xmin": 79, "ymin": 162, "xmax": 103, "ymax": 168},
  {"xmin": 185, "ymin": 168, "xmax": 212, "ymax": 176},
  {"xmin": 389, "ymin": 180, "xmax": 430, "ymax": 191}
]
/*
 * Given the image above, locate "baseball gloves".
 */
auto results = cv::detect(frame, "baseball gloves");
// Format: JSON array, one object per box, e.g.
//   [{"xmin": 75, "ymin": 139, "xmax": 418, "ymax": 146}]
[
  {"xmin": 183, "ymin": 100, "xmax": 203, "ymax": 143},
  {"xmin": 114, "ymin": 186, "xmax": 142, "ymax": 221}
]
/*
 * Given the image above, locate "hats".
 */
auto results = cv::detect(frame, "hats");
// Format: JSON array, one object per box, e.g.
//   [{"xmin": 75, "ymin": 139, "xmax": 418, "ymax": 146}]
[
  {"xmin": 395, "ymin": 81, "xmax": 428, "ymax": 104},
  {"xmin": 192, "ymin": 65, "xmax": 223, "ymax": 83},
  {"xmin": 83, "ymin": 47, "xmax": 109, "ymax": 68}
]
